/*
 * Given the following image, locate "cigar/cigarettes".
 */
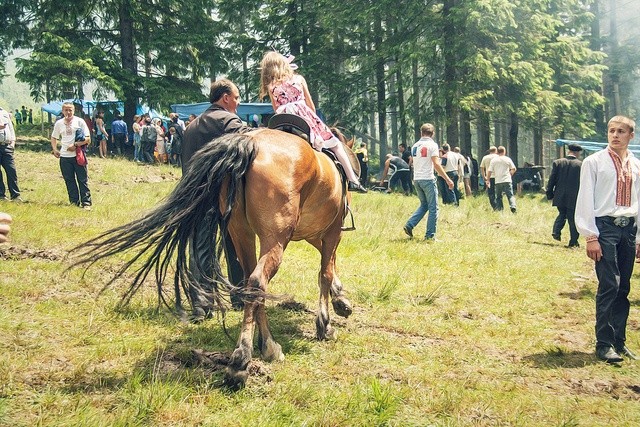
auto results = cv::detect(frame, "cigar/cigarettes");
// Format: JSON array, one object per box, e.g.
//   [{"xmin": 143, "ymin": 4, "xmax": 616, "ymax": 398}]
[{"xmin": 66, "ymin": 115, "xmax": 69, "ymax": 119}]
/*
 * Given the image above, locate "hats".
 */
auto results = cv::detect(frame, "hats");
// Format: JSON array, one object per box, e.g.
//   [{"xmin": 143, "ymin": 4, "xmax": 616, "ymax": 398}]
[{"xmin": 568, "ymin": 143, "xmax": 582, "ymax": 150}]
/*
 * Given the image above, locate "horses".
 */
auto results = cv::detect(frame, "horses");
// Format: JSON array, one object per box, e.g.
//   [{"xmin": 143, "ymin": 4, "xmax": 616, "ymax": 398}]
[{"xmin": 58, "ymin": 120, "xmax": 355, "ymax": 392}]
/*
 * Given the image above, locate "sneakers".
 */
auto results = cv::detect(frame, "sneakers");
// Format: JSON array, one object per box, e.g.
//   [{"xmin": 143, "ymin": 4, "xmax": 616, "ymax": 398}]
[{"xmin": 404, "ymin": 226, "xmax": 413, "ymax": 237}]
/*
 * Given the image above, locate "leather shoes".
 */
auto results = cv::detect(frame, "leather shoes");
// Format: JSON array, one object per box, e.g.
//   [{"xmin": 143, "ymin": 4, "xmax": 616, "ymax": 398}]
[
  {"xmin": 597, "ymin": 346, "xmax": 623, "ymax": 363},
  {"xmin": 616, "ymin": 346, "xmax": 636, "ymax": 360},
  {"xmin": 347, "ymin": 180, "xmax": 369, "ymax": 194}
]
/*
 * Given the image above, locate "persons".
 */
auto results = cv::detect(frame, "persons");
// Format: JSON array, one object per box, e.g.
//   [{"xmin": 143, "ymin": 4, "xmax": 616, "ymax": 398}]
[
  {"xmin": 51, "ymin": 102, "xmax": 92, "ymax": 212},
  {"xmin": 468, "ymin": 154, "xmax": 478, "ymax": 195},
  {"xmin": 574, "ymin": 116, "xmax": 640, "ymax": 362},
  {"xmin": 84, "ymin": 114, "xmax": 92, "ymax": 135},
  {"xmin": 8, "ymin": 111, "xmax": 14, "ymax": 126},
  {"xmin": 145, "ymin": 113, "xmax": 150, "ymax": 122},
  {"xmin": 169, "ymin": 127, "xmax": 181, "ymax": 167},
  {"xmin": 111, "ymin": 115, "xmax": 129, "ymax": 155},
  {"xmin": 0, "ymin": 212, "xmax": 12, "ymax": 243},
  {"xmin": 487, "ymin": 146, "xmax": 517, "ymax": 213},
  {"xmin": 357, "ymin": 142, "xmax": 367, "ymax": 188},
  {"xmin": 29, "ymin": 109, "xmax": 33, "ymax": 125},
  {"xmin": 133, "ymin": 115, "xmax": 140, "ymax": 162},
  {"xmin": 452, "ymin": 146, "xmax": 467, "ymax": 199},
  {"xmin": 403, "ymin": 123, "xmax": 454, "ymax": 240},
  {"xmin": 15, "ymin": 109, "xmax": 21, "ymax": 124},
  {"xmin": 188, "ymin": 114, "xmax": 196, "ymax": 121},
  {"xmin": 0, "ymin": 106, "xmax": 20, "ymax": 201},
  {"xmin": 252, "ymin": 115, "xmax": 259, "ymax": 127},
  {"xmin": 113, "ymin": 110, "xmax": 119, "ymax": 119},
  {"xmin": 182, "ymin": 80, "xmax": 246, "ymax": 318},
  {"xmin": 170, "ymin": 112, "xmax": 186, "ymax": 130},
  {"xmin": 185, "ymin": 121, "xmax": 190, "ymax": 125},
  {"xmin": 21, "ymin": 106, "xmax": 28, "ymax": 123},
  {"xmin": 436, "ymin": 149, "xmax": 445, "ymax": 205},
  {"xmin": 440, "ymin": 142, "xmax": 458, "ymax": 206},
  {"xmin": 171, "ymin": 113, "xmax": 186, "ymax": 136},
  {"xmin": 96, "ymin": 110, "xmax": 109, "ymax": 158},
  {"xmin": 143, "ymin": 119, "xmax": 156, "ymax": 165},
  {"xmin": 160, "ymin": 120, "xmax": 166, "ymax": 131},
  {"xmin": 399, "ymin": 144, "xmax": 413, "ymax": 197},
  {"xmin": 380, "ymin": 154, "xmax": 410, "ymax": 195},
  {"xmin": 141, "ymin": 115, "xmax": 146, "ymax": 162},
  {"xmin": 151, "ymin": 118, "xmax": 165, "ymax": 164},
  {"xmin": 260, "ymin": 51, "xmax": 367, "ymax": 191},
  {"xmin": 546, "ymin": 144, "xmax": 583, "ymax": 247},
  {"xmin": 480, "ymin": 146, "xmax": 497, "ymax": 209},
  {"xmin": 463, "ymin": 152, "xmax": 472, "ymax": 196}
]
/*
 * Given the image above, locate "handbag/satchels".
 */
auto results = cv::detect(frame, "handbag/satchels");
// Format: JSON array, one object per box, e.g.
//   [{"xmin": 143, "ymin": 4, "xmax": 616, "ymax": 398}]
[{"xmin": 97, "ymin": 132, "xmax": 105, "ymax": 140}]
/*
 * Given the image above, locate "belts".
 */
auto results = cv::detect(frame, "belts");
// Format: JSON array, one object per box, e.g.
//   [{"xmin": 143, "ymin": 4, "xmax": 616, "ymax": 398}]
[{"xmin": 598, "ymin": 216, "xmax": 637, "ymax": 227}]
